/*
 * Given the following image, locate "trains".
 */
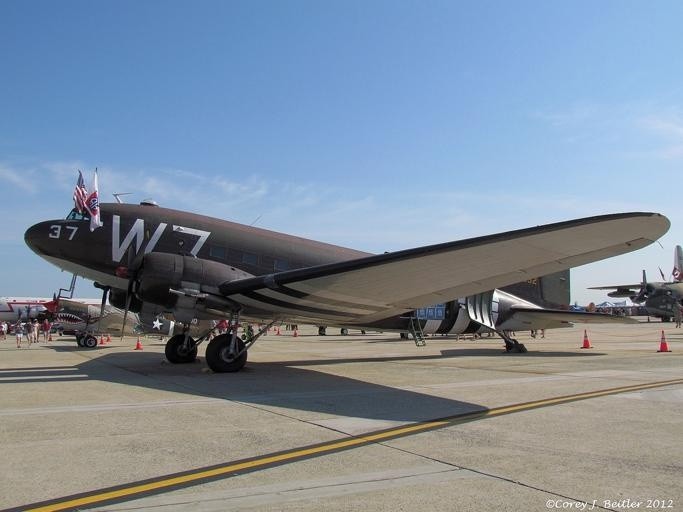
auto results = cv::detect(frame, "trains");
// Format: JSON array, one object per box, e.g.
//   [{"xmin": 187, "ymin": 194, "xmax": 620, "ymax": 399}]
[
  {"xmin": 586, "ymin": 245, "xmax": 683, "ymax": 322},
  {"xmin": 24, "ymin": 192, "xmax": 670, "ymax": 374}
]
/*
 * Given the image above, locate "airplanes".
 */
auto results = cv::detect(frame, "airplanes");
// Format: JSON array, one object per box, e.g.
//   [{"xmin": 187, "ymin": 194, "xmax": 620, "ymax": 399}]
[{"xmin": 0, "ymin": 297, "xmax": 145, "ymax": 348}]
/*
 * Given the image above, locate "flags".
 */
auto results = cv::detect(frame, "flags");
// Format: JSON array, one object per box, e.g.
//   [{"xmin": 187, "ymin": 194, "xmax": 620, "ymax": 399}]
[
  {"xmin": 73, "ymin": 173, "xmax": 87, "ymax": 213},
  {"xmin": 671, "ymin": 266, "xmax": 682, "ymax": 283},
  {"xmin": 83, "ymin": 173, "xmax": 102, "ymax": 232}
]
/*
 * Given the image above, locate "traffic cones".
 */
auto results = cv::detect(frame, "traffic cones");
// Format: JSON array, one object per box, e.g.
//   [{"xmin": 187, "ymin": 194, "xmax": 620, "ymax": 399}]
[
  {"xmin": 657, "ymin": 329, "xmax": 672, "ymax": 353},
  {"xmin": 98, "ymin": 331, "xmax": 112, "ymax": 345},
  {"xmin": 580, "ymin": 329, "xmax": 593, "ymax": 349},
  {"xmin": 134, "ymin": 335, "xmax": 143, "ymax": 351},
  {"xmin": 268, "ymin": 324, "xmax": 299, "ymax": 338},
  {"xmin": 48, "ymin": 334, "xmax": 53, "ymax": 341}
]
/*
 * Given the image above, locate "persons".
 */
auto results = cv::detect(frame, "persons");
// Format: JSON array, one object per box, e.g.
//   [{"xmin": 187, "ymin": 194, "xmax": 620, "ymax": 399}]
[
  {"xmin": 0, "ymin": 318, "xmax": 51, "ymax": 348},
  {"xmin": 672, "ymin": 301, "xmax": 682, "ymax": 328}
]
